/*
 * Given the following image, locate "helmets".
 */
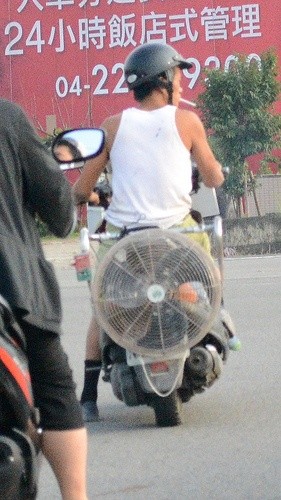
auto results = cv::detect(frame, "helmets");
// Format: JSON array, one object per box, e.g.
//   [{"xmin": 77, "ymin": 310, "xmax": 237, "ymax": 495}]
[{"xmin": 125, "ymin": 42, "xmax": 193, "ymax": 90}]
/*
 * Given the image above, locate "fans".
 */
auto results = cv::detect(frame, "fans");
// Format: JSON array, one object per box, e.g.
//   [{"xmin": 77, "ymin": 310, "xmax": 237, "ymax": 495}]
[{"xmin": 89, "ymin": 228, "xmax": 222, "ymax": 356}]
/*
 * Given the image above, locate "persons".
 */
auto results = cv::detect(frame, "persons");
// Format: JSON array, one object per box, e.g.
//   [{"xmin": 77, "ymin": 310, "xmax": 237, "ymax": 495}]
[
  {"xmin": 0, "ymin": 98, "xmax": 87, "ymax": 500},
  {"xmin": 72, "ymin": 42, "xmax": 241, "ymax": 421},
  {"xmin": 54, "ymin": 138, "xmax": 83, "ymax": 161}
]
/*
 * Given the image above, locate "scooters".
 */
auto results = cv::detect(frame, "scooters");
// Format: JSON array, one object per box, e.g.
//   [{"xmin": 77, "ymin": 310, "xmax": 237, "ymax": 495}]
[
  {"xmin": 93, "ymin": 166, "xmax": 242, "ymax": 429},
  {"xmin": 0, "ymin": 128, "xmax": 106, "ymax": 500}
]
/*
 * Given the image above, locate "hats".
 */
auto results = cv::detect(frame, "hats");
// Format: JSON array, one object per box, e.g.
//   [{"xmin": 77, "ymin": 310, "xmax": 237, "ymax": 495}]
[{"xmin": 57, "ymin": 138, "xmax": 82, "ymax": 159}]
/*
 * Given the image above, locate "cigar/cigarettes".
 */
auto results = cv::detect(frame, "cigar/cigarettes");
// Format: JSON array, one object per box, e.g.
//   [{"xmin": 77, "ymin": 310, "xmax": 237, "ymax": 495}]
[{"xmin": 181, "ymin": 98, "xmax": 196, "ymax": 107}]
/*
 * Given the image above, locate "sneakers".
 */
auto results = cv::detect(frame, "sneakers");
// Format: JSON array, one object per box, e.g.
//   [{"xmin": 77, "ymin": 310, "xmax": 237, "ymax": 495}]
[{"xmin": 79, "ymin": 401, "xmax": 99, "ymax": 422}]
